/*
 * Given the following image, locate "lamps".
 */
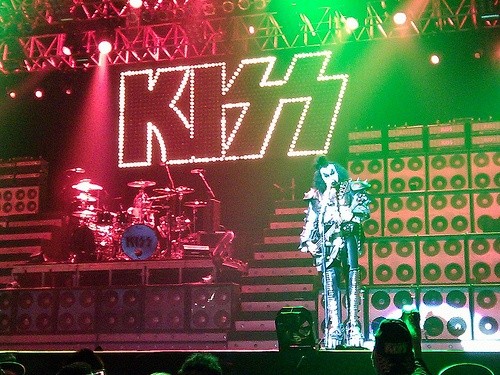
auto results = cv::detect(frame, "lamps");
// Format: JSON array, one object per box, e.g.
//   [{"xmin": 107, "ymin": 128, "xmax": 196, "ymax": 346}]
[
  {"xmin": 62, "ymin": 36, "xmax": 78, "ymax": 55},
  {"xmin": 98, "ymin": 36, "xmax": 112, "ymax": 53}
]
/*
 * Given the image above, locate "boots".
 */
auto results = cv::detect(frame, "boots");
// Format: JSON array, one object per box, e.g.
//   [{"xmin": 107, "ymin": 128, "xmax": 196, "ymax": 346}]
[
  {"xmin": 346, "ymin": 267, "xmax": 363, "ymax": 347},
  {"xmin": 324, "ymin": 269, "xmax": 344, "ymax": 346}
]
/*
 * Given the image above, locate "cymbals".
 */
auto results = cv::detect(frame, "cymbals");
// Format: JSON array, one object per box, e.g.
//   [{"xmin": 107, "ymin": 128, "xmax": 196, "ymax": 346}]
[
  {"xmin": 74, "ymin": 196, "xmax": 98, "ymax": 202},
  {"xmin": 127, "ymin": 180, "xmax": 156, "ymax": 188},
  {"xmin": 183, "ymin": 201, "xmax": 208, "ymax": 207},
  {"xmin": 72, "ymin": 182, "xmax": 104, "ymax": 193},
  {"xmin": 153, "ymin": 188, "xmax": 195, "ymax": 195}
]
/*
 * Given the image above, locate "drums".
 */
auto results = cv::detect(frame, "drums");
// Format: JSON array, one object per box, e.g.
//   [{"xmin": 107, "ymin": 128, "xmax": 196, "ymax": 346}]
[{"xmin": 96, "ymin": 212, "xmax": 189, "ymax": 260}]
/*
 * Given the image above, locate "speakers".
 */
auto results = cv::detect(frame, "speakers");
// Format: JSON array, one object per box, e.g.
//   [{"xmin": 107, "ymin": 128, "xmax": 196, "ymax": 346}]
[
  {"xmin": 58, "ymin": 288, "xmax": 99, "ymax": 334},
  {"xmin": 189, "ymin": 283, "xmax": 241, "ymax": 332},
  {"xmin": 345, "ymin": 150, "xmax": 500, "ymax": 342},
  {"xmin": 203, "ymin": 199, "xmax": 223, "ymax": 233},
  {"xmin": 96, "ymin": 285, "xmax": 145, "ymax": 335},
  {"xmin": 0, "ymin": 288, "xmax": 18, "ymax": 335},
  {"xmin": 143, "ymin": 283, "xmax": 190, "ymax": 333},
  {"xmin": 275, "ymin": 306, "xmax": 319, "ymax": 352},
  {"xmin": 13, "ymin": 287, "xmax": 60, "ymax": 336}
]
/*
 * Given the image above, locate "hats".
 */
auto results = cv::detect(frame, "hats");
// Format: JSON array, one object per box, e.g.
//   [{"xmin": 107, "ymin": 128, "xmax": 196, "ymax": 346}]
[{"xmin": 0, "ymin": 353, "xmax": 25, "ymax": 375}]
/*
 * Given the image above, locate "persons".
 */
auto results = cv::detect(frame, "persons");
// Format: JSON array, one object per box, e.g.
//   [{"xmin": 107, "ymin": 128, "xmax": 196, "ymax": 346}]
[
  {"xmin": 300, "ymin": 155, "xmax": 373, "ymax": 348},
  {"xmin": 125, "ymin": 194, "xmax": 157, "ymax": 228},
  {"xmin": 1, "ymin": 310, "xmax": 428, "ymax": 375}
]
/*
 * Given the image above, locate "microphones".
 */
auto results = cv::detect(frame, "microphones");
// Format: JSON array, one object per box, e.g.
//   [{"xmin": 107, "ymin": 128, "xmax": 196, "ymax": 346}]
[
  {"xmin": 327, "ymin": 180, "xmax": 336, "ymax": 196},
  {"xmin": 159, "ymin": 163, "xmax": 173, "ymax": 168},
  {"xmin": 191, "ymin": 169, "xmax": 205, "ymax": 173},
  {"xmin": 67, "ymin": 168, "xmax": 86, "ymax": 174}
]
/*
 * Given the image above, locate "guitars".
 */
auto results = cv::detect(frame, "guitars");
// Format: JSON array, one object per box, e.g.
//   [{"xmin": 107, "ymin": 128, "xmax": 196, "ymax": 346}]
[{"xmin": 308, "ymin": 192, "xmax": 364, "ymax": 272}]
